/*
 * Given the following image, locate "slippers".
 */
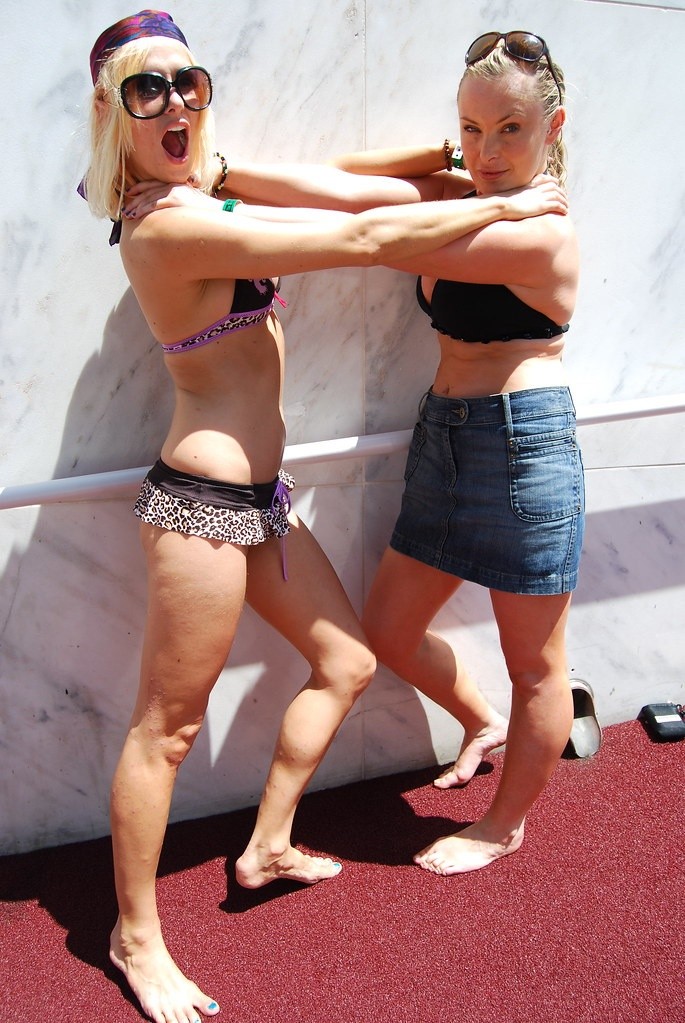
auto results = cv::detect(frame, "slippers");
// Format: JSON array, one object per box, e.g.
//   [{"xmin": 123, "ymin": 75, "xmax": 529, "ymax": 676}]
[{"xmin": 568, "ymin": 678, "xmax": 602, "ymax": 758}]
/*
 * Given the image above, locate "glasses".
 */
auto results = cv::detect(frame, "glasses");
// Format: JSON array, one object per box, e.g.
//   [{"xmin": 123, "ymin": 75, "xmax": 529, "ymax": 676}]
[
  {"xmin": 464, "ymin": 30, "xmax": 562, "ymax": 108},
  {"xmin": 97, "ymin": 66, "xmax": 214, "ymax": 121}
]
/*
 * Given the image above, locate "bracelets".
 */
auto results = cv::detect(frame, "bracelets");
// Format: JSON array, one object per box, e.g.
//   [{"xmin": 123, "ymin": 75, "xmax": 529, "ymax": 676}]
[
  {"xmin": 211, "ymin": 152, "xmax": 228, "ymax": 198},
  {"xmin": 442, "ymin": 137, "xmax": 454, "ymax": 171},
  {"xmin": 223, "ymin": 198, "xmax": 243, "ymax": 213},
  {"xmin": 451, "ymin": 146, "xmax": 467, "ymax": 170}
]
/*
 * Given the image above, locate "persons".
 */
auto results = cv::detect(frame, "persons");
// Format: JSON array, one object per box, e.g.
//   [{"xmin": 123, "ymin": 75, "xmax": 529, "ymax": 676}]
[
  {"xmin": 81, "ymin": 18, "xmax": 568, "ymax": 1022},
  {"xmin": 116, "ymin": 30, "xmax": 580, "ymax": 877}
]
